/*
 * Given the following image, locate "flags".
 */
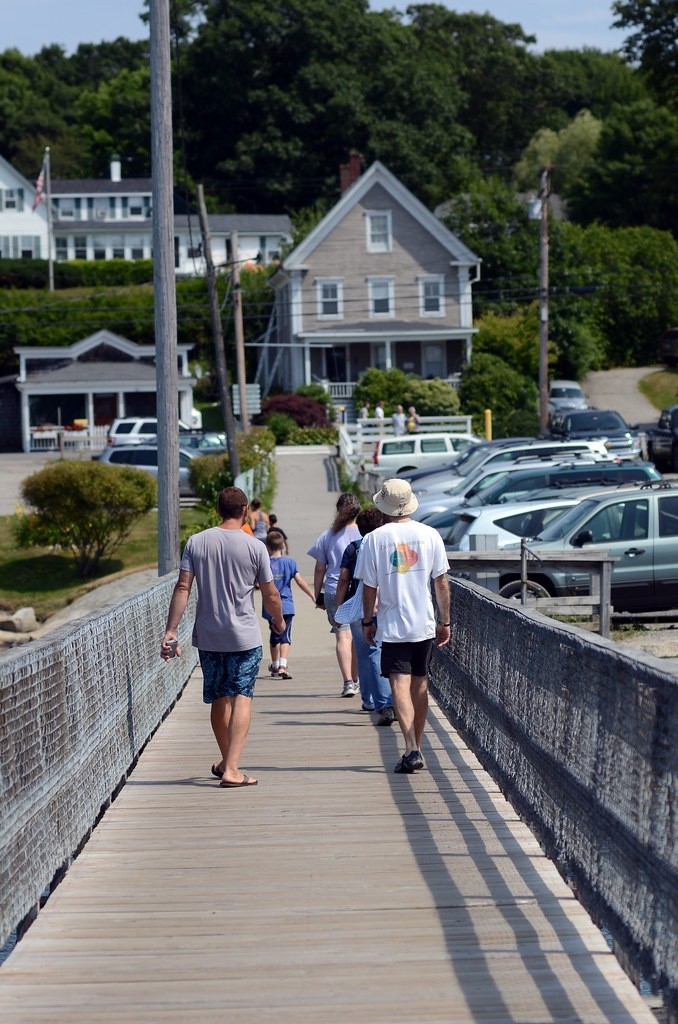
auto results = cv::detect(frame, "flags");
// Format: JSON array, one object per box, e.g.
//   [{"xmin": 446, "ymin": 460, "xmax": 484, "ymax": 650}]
[{"xmin": 33, "ymin": 165, "xmax": 45, "ymax": 208}]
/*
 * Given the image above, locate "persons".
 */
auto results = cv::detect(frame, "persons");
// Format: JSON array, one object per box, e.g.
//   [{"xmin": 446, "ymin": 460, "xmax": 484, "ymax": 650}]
[
  {"xmin": 307, "ymin": 493, "xmax": 363, "ymax": 696},
  {"xmin": 334, "ymin": 506, "xmax": 383, "ymax": 711},
  {"xmin": 353, "ymin": 479, "xmax": 450, "ymax": 774},
  {"xmin": 160, "ymin": 487, "xmax": 286, "ymax": 786},
  {"xmin": 375, "ymin": 401, "xmax": 384, "ymax": 418},
  {"xmin": 334, "ymin": 581, "xmax": 399, "ymax": 726},
  {"xmin": 392, "ymin": 405, "xmax": 405, "ymax": 437},
  {"xmin": 253, "ymin": 531, "xmax": 317, "ymax": 680},
  {"xmin": 267, "ymin": 515, "xmax": 288, "ymax": 556},
  {"xmin": 358, "ymin": 401, "xmax": 370, "ymax": 418},
  {"xmin": 242, "ymin": 499, "xmax": 269, "ymax": 590},
  {"xmin": 407, "ymin": 407, "xmax": 420, "ymax": 433}
]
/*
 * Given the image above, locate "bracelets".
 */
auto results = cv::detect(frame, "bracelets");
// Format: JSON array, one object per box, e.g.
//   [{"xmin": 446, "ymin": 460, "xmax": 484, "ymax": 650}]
[
  {"xmin": 361, "ymin": 619, "xmax": 373, "ymax": 627},
  {"xmin": 439, "ymin": 621, "xmax": 450, "ymax": 626}
]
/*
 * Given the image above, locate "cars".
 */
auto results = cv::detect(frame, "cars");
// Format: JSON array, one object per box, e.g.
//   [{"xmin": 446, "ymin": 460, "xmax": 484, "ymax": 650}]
[{"xmin": 391, "ymin": 406, "xmax": 678, "ymax": 580}]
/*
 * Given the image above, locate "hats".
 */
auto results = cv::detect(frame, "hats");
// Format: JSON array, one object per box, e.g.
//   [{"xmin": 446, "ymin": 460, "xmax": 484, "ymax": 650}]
[{"xmin": 372, "ymin": 479, "xmax": 418, "ymax": 517}]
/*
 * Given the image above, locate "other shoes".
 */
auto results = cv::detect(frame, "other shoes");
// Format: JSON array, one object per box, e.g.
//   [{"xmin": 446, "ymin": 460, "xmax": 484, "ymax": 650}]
[
  {"xmin": 361, "ymin": 704, "xmax": 374, "ymax": 711},
  {"xmin": 376, "ymin": 709, "xmax": 398, "ymax": 726},
  {"xmin": 268, "ymin": 664, "xmax": 279, "ymax": 677},
  {"xmin": 254, "ymin": 584, "xmax": 260, "ymax": 590},
  {"xmin": 277, "ymin": 665, "xmax": 293, "ymax": 679}
]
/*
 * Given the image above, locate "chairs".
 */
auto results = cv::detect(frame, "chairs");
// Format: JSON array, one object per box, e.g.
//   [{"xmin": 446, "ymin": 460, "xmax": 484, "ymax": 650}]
[
  {"xmin": 634, "ymin": 509, "xmax": 648, "ymax": 539},
  {"xmin": 524, "ymin": 509, "xmax": 546, "ymax": 537}
]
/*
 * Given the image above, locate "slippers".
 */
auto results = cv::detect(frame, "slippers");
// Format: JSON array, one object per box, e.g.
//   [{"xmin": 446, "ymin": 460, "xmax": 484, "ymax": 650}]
[
  {"xmin": 211, "ymin": 763, "xmax": 223, "ymax": 778},
  {"xmin": 219, "ymin": 775, "xmax": 258, "ymax": 786}
]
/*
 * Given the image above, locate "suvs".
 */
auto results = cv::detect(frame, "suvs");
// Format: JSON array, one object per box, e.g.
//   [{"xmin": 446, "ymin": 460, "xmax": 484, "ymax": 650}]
[
  {"xmin": 96, "ymin": 443, "xmax": 204, "ymax": 498},
  {"xmin": 547, "ymin": 405, "xmax": 643, "ymax": 462},
  {"xmin": 371, "ymin": 432, "xmax": 515, "ymax": 480},
  {"xmin": 447, "ymin": 487, "xmax": 678, "ymax": 621},
  {"xmin": 107, "ymin": 415, "xmax": 226, "ymax": 449}
]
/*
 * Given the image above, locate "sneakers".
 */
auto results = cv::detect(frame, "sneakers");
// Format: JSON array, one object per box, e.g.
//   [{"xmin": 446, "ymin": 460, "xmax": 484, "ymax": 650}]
[
  {"xmin": 340, "ymin": 680, "xmax": 356, "ymax": 696},
  {"xmin": 354, "ymin": 677, "xmax": 360, "ymax": 693},
  {"xmin": 393, "ymin": 750, "xmax": 424, "ymax": 773}
]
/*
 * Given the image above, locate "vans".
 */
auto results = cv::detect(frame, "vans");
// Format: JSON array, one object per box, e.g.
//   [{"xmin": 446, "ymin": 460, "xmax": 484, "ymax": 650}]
[{"xmin": 545, "ymin": 379, "xmax": 592, "ymax": 418}]
